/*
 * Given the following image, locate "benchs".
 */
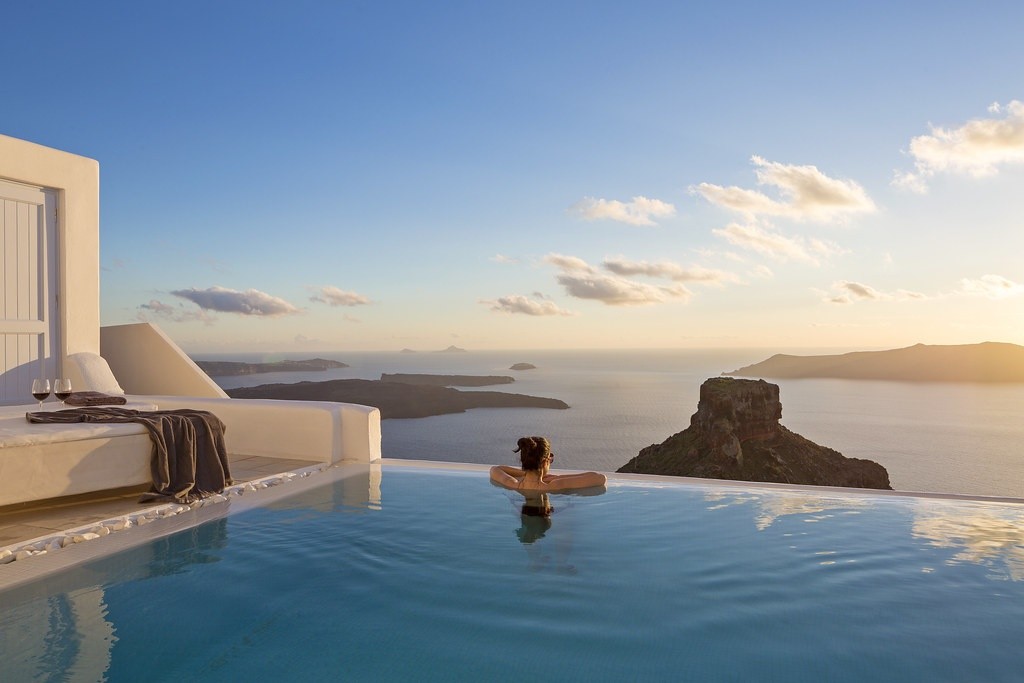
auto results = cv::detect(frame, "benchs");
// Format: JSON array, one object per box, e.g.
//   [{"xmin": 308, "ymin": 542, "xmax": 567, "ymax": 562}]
[
  {"xmin": 0, "ymin": 397, "xmax": 160, "ymax": 426},
  {"xmin": 1, "ymin": 412, "xmax": 223, "ymax": 511}
]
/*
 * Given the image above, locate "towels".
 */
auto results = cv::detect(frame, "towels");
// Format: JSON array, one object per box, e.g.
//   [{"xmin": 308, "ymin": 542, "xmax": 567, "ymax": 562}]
[
  {"xmin": 63, "ymin": 389, "xmax": 128, "ymax": 408},
  {"xmin": 25, "ymin": 405, "xmax": 236, "ymax": 506}
]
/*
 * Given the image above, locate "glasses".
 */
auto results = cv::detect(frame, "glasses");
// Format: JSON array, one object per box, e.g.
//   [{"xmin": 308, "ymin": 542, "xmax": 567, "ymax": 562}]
[{"xmin": 544, "ymin": 452, "xmax": 554, "ymax": 464}]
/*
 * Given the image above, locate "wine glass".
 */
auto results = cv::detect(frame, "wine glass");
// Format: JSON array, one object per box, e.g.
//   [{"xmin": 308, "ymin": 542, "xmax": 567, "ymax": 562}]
[
  {"xmin": 32, "ymin": 378, "xmax": 50, "ymax": 412},
  {"xmin": 54, "ymin": 378, "xmax": 72, "ymax": 412}
]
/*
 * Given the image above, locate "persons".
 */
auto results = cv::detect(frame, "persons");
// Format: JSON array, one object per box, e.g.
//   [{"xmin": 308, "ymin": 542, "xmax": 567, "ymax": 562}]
[{"xmin": 489, "ymin": 435, "xmax": 608, "ymax": 491}]
